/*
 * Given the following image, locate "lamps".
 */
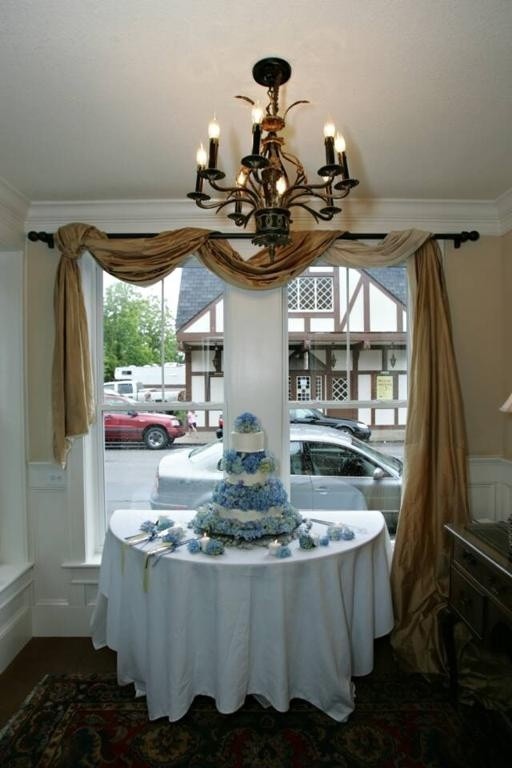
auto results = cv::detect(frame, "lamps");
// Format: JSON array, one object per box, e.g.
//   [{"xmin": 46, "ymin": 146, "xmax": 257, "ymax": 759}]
[{"xmin": 184, "ymin": 54, "xmax": 362, "ymax": 262}]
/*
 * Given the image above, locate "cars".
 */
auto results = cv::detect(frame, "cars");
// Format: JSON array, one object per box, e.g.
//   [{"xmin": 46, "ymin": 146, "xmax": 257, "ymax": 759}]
[
  {"xmin": 151, "ymin": 423, "xmax": 405, "ymax": 535},
  {"xmin": 104, "ymin": 393, "xmax": 185, "ymax": 450},
  {"xmin": 216, "ymin": 408, "xmax": 371, "ymax": 440}
]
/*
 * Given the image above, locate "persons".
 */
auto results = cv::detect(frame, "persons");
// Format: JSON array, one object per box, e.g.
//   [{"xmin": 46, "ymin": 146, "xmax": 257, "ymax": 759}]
[{"xmin": 186, "ymin": 410, "xmax": 199, "ymax": 438}]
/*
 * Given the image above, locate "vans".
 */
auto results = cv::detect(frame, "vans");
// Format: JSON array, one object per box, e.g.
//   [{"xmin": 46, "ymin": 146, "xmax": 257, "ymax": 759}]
[{"xmin": 102, "ymin": 380, "xmax": 144, "ymax": 403}]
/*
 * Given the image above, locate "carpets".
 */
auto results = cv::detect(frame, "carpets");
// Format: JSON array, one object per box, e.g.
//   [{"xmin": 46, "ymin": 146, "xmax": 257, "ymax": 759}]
[
  {"xmin": 0, "ymin": 669, "xmax": 512, "ymax": 768},
  {"xmin": 2, "ymin": 669, "xmax": 511, "ymax": 768}
]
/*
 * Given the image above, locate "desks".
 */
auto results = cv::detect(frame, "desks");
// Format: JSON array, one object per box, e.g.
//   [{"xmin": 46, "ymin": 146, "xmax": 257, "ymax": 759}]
[
  {"xmin": 108, "ymin": 506, "xmax": 387, "ymax": 722},
  {"xmin": 435, "ymin": 519, "xmax": 512, "ymax": 768},
  {"xmin": 435, "ymin": 519, "xmax": 510, "ymax": 768}
]
